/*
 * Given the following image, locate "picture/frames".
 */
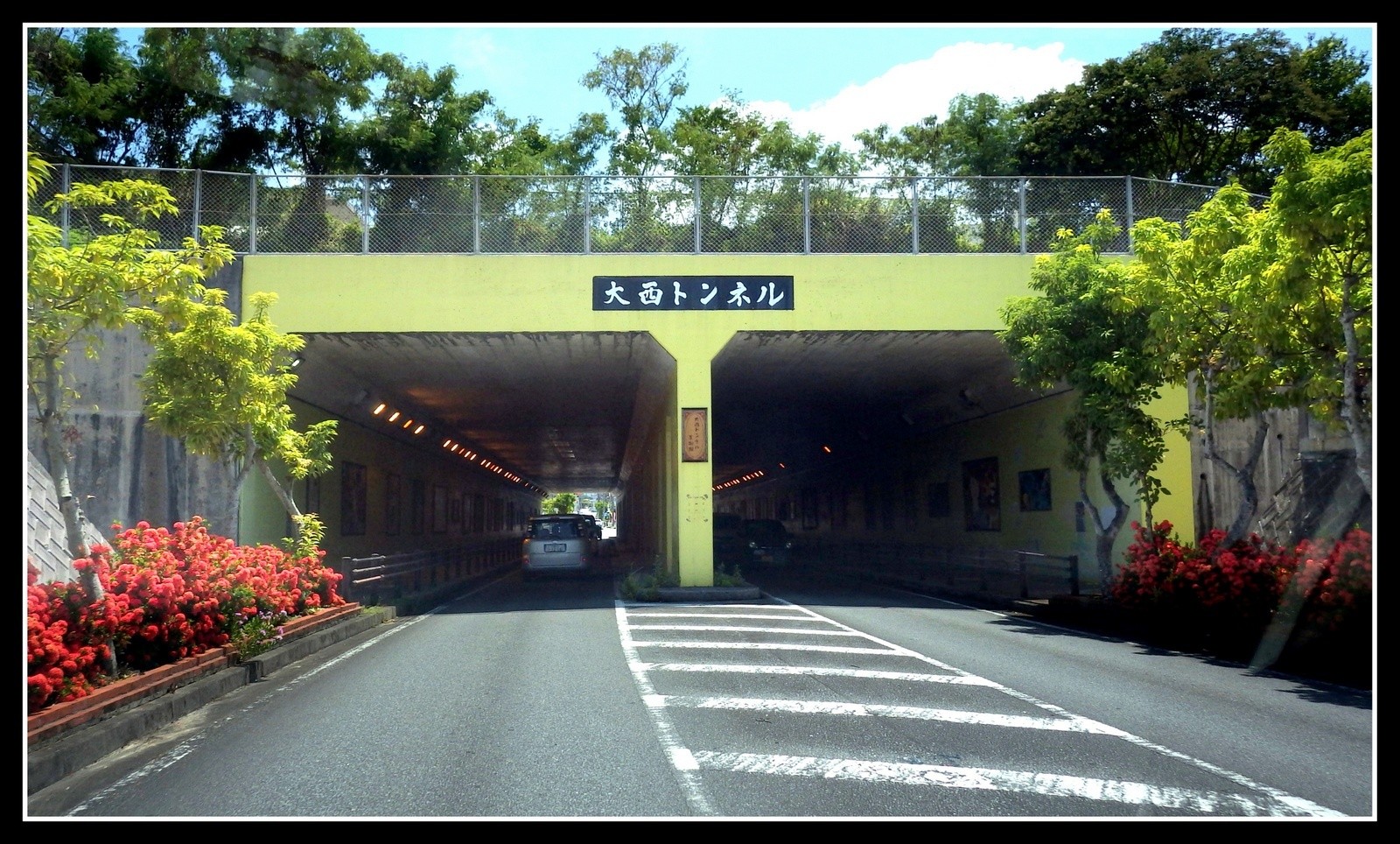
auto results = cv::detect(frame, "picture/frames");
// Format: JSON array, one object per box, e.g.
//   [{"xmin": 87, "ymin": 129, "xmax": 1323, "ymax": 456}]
[
  {"xmin": 773, "ymin": 455, "xmax": 1052, "ymax": 533},
  {"xmin": 306, "ymin": 460, "xmax": 501, "ymax": 537}
]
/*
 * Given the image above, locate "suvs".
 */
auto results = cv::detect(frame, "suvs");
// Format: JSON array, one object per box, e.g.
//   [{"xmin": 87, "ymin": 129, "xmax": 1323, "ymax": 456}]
[
  {"xmin": 520, "ymin": 514, "xmax": 604, "ymax": 591},
  {"xmin": 734, "ymin": 518, "xmax": 796, "ymax": 568}
]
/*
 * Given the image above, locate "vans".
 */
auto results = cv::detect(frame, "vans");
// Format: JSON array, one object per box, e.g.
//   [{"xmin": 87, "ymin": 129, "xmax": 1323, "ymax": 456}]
[{"xmin": 714, "ymin": 512, "xmax": 742, "ymax": 555}]
